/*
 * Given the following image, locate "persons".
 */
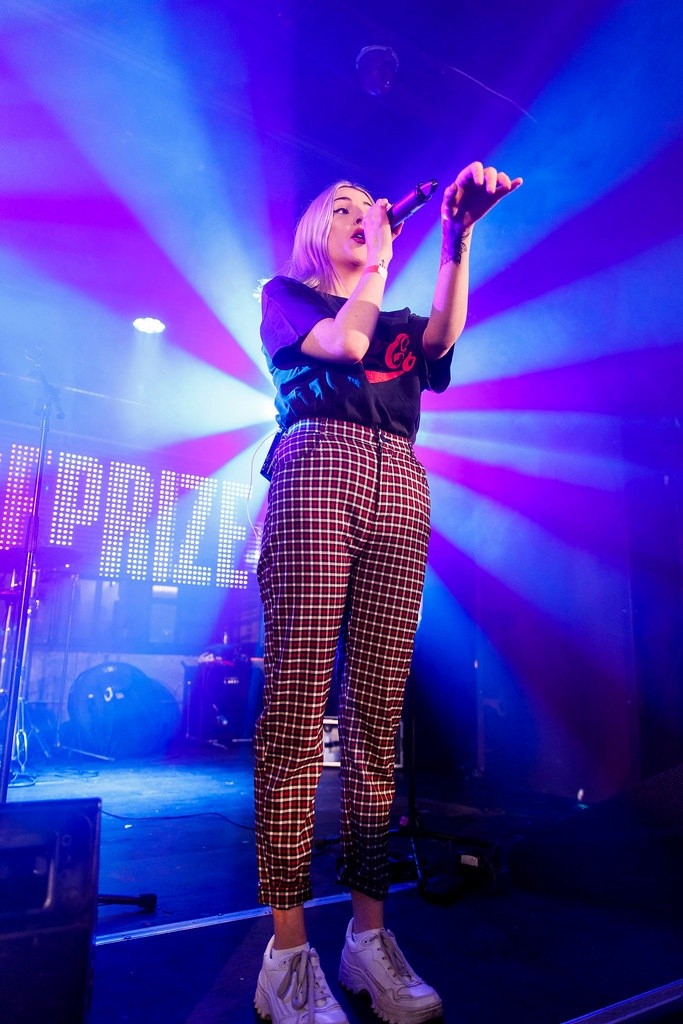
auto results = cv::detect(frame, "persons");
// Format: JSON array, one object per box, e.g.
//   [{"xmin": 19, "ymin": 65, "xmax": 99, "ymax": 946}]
[{"xmin": 253, "ymin": 157, "xmax": 527, "ymax": 1023}]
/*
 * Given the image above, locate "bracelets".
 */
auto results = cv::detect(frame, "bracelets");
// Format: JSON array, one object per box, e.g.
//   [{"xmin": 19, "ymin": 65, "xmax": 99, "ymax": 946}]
[{"xmin": 360, "ymin": 265, "xmax": 389, "ymax": 280}]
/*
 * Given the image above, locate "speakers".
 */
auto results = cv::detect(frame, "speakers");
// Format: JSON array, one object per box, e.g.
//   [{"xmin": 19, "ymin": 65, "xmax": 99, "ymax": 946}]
[
  {"xmin": 0, "ymin": 798, "xmax": 102, "ymax": 1024},
  {"xmin": 548, "ymin": 764, "xmax": 683, "ymax": 907}
]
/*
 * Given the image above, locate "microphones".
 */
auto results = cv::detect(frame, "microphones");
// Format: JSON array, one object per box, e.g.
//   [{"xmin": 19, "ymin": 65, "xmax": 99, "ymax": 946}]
[{"xmin": 386, "ymin": 180, "xmax": 438, "ymax": 230}]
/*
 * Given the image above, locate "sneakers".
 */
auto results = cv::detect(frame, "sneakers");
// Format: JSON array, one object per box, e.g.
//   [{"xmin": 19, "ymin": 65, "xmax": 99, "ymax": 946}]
[
  {"xmin": 254, "ymin": 935, "xmax": 350, "ymax": 1024},
  {"xmin": 338, "ymin": 916, "xmax": 443, "ymax": 1024}
]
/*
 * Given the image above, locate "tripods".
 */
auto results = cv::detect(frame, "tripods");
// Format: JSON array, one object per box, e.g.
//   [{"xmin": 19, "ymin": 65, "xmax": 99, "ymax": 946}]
[
  {"xmin": 314, "ymin": 670, "xmax": 500, "ymax": 892},
  {"xmin": 25, "ymin": 573, "xmax": 114, "ymax": 763}
]
